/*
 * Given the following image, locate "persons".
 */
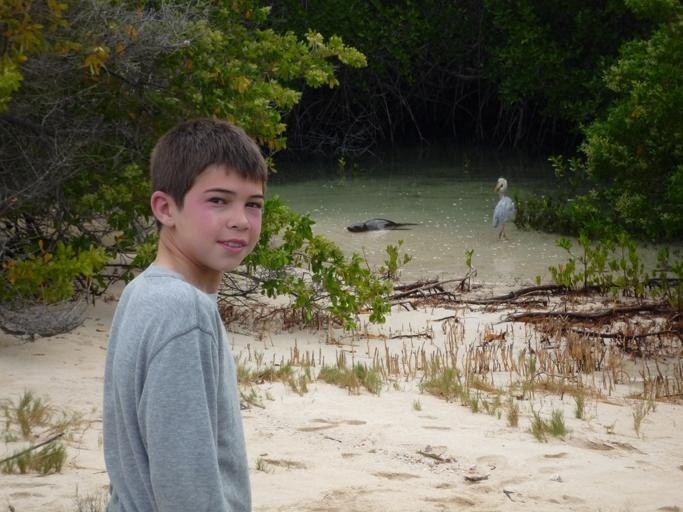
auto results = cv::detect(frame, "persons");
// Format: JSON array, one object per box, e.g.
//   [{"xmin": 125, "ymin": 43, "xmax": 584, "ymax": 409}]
[{"xmin": 102, "ymin": 115, "xmax": 268, "ymax": 512}]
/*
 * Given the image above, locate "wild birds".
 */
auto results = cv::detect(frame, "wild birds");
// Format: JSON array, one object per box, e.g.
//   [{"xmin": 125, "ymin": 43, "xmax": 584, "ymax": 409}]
[
  {"xmin": 347, "ymin": 218, "xmax": 419, "ymax": 232},
  {"xmin": 492, "ymin": 177, "xmax": 517, "ymax": 240}
]
